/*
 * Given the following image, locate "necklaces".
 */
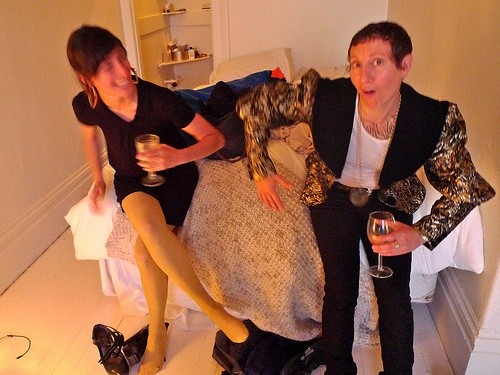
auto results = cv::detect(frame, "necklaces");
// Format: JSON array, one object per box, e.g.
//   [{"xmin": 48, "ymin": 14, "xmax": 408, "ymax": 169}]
[{"xmin": 351, "ymin": 91, "xmax": 402, "ymax": 206}]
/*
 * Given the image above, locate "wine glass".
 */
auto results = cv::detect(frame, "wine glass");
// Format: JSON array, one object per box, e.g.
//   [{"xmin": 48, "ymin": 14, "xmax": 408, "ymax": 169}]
[
  {"xmin": 135, "ymin": 134, "xmax": 165, "ymax": 187},
  {"xmin": 367, "ymin": 211, "xmax": 395, "ymax": 278}
]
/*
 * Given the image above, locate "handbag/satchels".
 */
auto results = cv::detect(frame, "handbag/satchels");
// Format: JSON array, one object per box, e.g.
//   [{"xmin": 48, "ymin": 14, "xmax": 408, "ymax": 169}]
[{"xmin": 194, "ymin": 111, "xmax": 247, "ymax": 164}]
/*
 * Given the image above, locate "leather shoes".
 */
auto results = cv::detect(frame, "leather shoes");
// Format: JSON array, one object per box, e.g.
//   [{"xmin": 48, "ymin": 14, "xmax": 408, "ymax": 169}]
[
  {"xmin": 123, "ymin": 322, "xmax": 169, "ymax": 366},
  {"xmin": 92, "ymin": 324, "xmax": 129, "ymax": 375}
]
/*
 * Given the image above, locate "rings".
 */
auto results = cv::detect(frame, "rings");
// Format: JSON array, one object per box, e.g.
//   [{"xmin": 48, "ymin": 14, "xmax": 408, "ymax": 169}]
[{"xmin": 392, "ymin": 240, "xmax": 400, "ymax": 249}]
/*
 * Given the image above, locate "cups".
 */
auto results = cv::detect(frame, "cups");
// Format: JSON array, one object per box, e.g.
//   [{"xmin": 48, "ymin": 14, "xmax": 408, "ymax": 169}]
[{"xmin": 162, "ymin": 50, "xmax": 172, "ymax": 63}]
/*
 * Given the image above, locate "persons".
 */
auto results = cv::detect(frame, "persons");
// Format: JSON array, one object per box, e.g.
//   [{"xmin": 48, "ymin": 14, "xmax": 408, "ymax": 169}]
[
  {"xmin": 234, "ymin": 20, "xmax": 497, "ymax": 375},
  {"xmin": 65, "ymin": 21, "xmax": 251, "ymax": 375}
]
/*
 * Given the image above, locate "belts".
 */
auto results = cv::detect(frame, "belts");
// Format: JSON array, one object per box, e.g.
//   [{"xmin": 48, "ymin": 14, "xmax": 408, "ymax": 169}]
[{"xmin": 332, "ymin": 182, "xmax": 380, "ymax": 195}]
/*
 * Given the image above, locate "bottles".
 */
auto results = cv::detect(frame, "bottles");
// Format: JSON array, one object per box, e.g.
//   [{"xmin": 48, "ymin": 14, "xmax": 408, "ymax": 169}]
[
  {"xmin": 188, "ymin": 47, "xmax": 194, "ymax": 60},
  {"xmin": 165, "ymin": 5, "xmax": 170, "ymax": 13},
  {"xmin": 167, "ymin": 41, "xmax": 177, "ymax": 55}
]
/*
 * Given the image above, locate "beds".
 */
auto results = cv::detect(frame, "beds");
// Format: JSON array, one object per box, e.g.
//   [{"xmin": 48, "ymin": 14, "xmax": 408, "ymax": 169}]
[{"xmin": 64, "ymin": 47, "xmax": 484, "ymax": 346}]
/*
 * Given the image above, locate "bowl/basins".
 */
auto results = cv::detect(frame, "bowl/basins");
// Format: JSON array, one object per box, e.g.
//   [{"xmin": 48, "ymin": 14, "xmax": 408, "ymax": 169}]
[{"xmin": 173, "ymin": 50, "xmax": 188, "ymax": 62}]
